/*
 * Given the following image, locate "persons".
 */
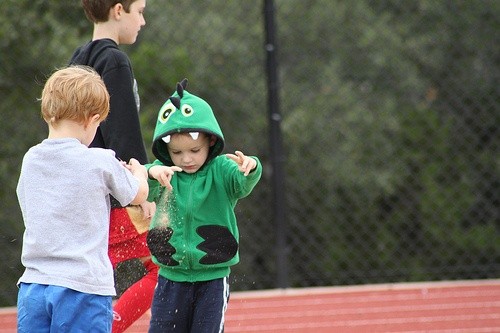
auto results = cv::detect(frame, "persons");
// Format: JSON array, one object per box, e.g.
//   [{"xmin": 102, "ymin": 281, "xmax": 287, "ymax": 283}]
[
  {"xmin": 65, "ymin": 0, "xmax": 161, "ymax": 333},
  {"xmin": 14, "ymin": 65, "xmax": 148, "ymax": 333},
  {"xmin": 125, "ymin": 78, "xmax": 262, "ymax": 333}
]
